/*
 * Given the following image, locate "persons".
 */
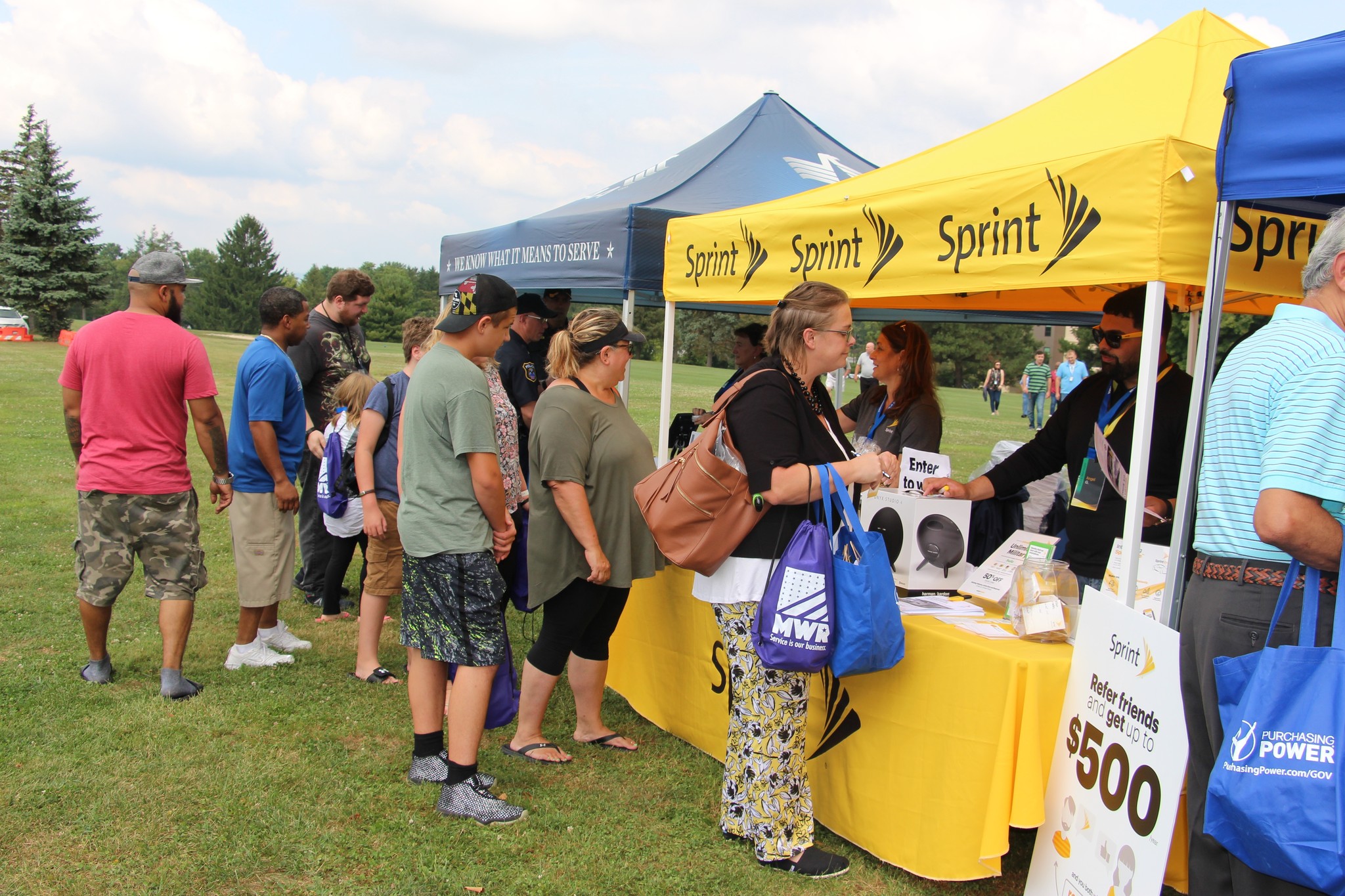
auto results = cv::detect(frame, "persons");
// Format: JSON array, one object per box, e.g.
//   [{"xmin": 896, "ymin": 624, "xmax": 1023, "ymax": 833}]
[
  {"xmin": 837, "ymin": 318, "xmax": 943, "ymax": 488},
  {"xmin": 58, "ymin": 251, "xmax": 234, "ymax": 700},
  {"xmin": 397, "ymin": 274, "xmax": 529, "ymax": 825},
  {"xmin": 315, "ymin": 372, "xmax": 397, "ymax": 623},
  {"xmin": 225, "ymin": 286, "xmax": 312, "ymax": 670},
  {"xmin": 825, "ymin": 358, "xmax": 851, "ymax": 399},
  {"xmin": 502, "ymin": 306, "xmax": 673, "ymax": 761},
  {"xmin": 692, "ymin": 323, "xmax": 769, "ymax": 425},
  {"xmin": 689, "ymin": 281, "xmax": 900, "ymax": 878},
  {"xmin": 529, "ymin": 289, "xmax": 572, "ymax": 397},
  {"xmin": 397, "ymin": 300, "xmax": 529, "ymax": 716},
  {"xmin": 1179, "ymin": 207, "xmax": 1345, "ymax": 896},
  {"xmin": 493, "ymin": 292, "xmax": 558, "ymax": 483},
  {"xmin": 922, "ymin": 283, "xmax": 1205, "ymax": 605},
  {"xmin": 348, "ymin": 317, "xmax": 437, "ymax": 685},
  {"xmin": 983, "ymin": 360, "xmax": 1004, "ymax": 415},
  {"xmin": 854, "ymin": 342, "xmax": 882, "ymax": 394},
  {"xmin": 1019, "ymin": 350, "xmax": 1089, "ymax": 430},
  {"xmin": 288, "ymin": 269, "xmax": 376, "ymax": 609}
]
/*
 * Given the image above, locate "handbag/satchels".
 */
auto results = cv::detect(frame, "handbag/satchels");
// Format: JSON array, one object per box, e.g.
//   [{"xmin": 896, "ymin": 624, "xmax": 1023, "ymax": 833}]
[
  {"xmin": 751, "ymin": 462, "xmax": 904, "ymax": 679},
  {"xmin": 633, "ymin": 369, "xmax": 793, "ymax": 578},
  {"xmin": 1202, "ymin": 516, "xmax": 1345, "ymax": 896},
  {"xmin": 333, "ymin": 376, "xmax": 394, "ymax": 496},
  {"xmin": 983, "ymin": 386, "xmax": 988, "ymax": 402}
]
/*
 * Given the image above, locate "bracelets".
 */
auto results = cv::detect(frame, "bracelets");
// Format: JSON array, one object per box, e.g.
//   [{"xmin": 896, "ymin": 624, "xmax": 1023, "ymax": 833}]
[
  {"xmin": 542, "ymin": 380, "xmax": 547, "ymax": 390},
  {"xmin": 359, "ymin": 489, "xmax": 374, "ymax": 496},
  {"xmin": 520, "ymin": 489, "xmax": 529, "ymax": 506}
]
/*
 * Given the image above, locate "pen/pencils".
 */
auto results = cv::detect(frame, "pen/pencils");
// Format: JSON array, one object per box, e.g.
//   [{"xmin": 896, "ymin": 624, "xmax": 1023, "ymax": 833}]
[
  {"xmin": 939, "ymin": 485, "xmax": 948, "ymax": 494},
  {"xmin": 850, "ymin": 450, "xmax": 891, "ymax": 479}
]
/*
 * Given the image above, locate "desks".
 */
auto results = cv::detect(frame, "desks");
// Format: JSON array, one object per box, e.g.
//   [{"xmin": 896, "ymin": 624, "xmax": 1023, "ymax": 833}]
[{"xmin": 601, "ymin": 555, "xmax": 1190, "ymax": 896}]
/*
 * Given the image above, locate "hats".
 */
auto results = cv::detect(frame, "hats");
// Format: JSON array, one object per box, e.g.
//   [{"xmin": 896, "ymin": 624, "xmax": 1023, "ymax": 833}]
[
  {"xmin": 433, "ymin": 273, "xmax": 517, "ymax": 334},
  {"xmin": 128, "ymin": 251, "xmax": 204, "ymax": 284},
  {"xmin": 578, "ymin": 320, "xmax": 645, "ymax": 354},
  {"xmin": 544, "ymin": 289, "xmax": 572, "ymax": 301},
  {"xmin": 516, "ymin": 293, "xmax": 558, "ymax": 319}
]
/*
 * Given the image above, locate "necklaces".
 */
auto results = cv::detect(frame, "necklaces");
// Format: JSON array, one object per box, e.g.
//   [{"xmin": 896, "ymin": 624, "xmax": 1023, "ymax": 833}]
[
  {"xmin": 260, "ymin": 334, "xmax": 295, "ymax": 367},
  {"xmin": 779, "ymin": 353, "xmax": 823, "ymax": 415}
]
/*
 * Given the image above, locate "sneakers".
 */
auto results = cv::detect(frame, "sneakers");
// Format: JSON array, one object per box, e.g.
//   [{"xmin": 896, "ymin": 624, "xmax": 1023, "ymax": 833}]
[
  {"xmin": 406, "ymin": 747, "xmax": 497, "ymax": 791},
  {"xmin": 224, "ymin": 634, "xmax": 294, "ymax": 671},
  {"xmin": 305, "ymin": 592, "xmax": 355, "ymax": 609},
  {"xmin": 293, "ymin": 568, "xmax": 349, "ymax": 599},
  {"xmin": 436, "ymin": 774, "xmax": 528, "ymax": 827},
  {"xmin": 257, "ymin": 619, "xmax": 312, "ymax": 651}
]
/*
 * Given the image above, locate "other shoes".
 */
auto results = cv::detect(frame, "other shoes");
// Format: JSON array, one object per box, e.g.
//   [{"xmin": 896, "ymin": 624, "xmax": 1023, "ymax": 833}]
[{"xmin": 723, "ymin": 830, "xmax": 850, "ymax": 878}]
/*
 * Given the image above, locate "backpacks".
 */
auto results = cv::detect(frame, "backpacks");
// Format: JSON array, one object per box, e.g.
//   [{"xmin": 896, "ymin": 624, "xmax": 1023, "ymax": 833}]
[{"xmin": 316, "ymin": 432, "xmax": 348, "ymax": 518}]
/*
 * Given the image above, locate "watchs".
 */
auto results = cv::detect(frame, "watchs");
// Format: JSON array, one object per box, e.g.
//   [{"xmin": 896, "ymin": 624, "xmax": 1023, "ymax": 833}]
[
  {"xmin": 305, "ymin": 427, "xmax": 317, "ymax": 441},
  {"xmin": 212, "ymin": 470, "xmax": 234, "ymax": 484},
  {"xmin": 1153, "ymin": 499, "xmax": 1172, "ymax": 526}
]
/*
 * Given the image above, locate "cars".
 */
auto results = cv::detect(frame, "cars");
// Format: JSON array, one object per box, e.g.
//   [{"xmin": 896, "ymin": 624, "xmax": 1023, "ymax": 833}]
[
  {"xmin": 0, "ymin": 306, "xmax": 30, "ymax": 335},
  {"xmin": 848, "ymin": 373, "xmax": 860, "ymax": 380}
]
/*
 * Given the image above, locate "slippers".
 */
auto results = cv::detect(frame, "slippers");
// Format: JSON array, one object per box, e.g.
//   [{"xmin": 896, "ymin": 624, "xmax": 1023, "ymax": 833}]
[
  {"xmin": 348, "ymin": 667, "xmax": 400, "ymax": 684},
  {"xmin": 315, "ymin": 612, "xmax": 352, "ymax": 622},
  {"xmin": 159, "ymin": 679, "xmax": 203, "ymax": 702},
  {"xmin": 357, "ymin": 615, "xmax": 394, "ymax": 622},
  {"xmin": 570, "ymin": 732, "xmax": 639, "ymax": 751},
  {"xmin": 80, "ymin": 665, "xmax": 112, "ymax": 684},
  {"xmin": 501, "ymin": 742, "xmax": 573, "ymax": 765}
]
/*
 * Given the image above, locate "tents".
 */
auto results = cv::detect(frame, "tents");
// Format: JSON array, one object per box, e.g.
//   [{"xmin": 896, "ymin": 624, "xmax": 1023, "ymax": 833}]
[
  {"xmin": 1161, "ymin": 31, "xmax": 1345, "ymax": 632},
  {"xmin": 654, "ymin": 7, "xmax": 1327, "ymax": 609},
  {"xmin": 440, "ymin": 90, "xmax": 882, "ymax": 466}
]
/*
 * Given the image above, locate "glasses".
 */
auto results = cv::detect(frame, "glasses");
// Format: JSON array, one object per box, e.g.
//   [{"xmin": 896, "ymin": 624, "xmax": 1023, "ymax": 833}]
[
  {"xmin": 526, "ymin": 315, "xmax": 548, "ymax": 323},
  {"xmin": 996, "ymin": 364, "xmax": 1000, "ymax": 365},
  {"xmin": 615, "ymin": 344, "xmax": 634, "ymax": 355},
  {"xmin": 812, "ymin": 326, "xmax": 855, "ymax": 342},
  {"xmin": 1092, "ymin": 324, "xmax": 1143, "ymax": 349}
]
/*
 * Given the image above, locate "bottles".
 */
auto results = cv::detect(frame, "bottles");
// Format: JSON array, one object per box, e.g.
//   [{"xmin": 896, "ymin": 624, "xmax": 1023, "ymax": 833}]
[{"xmin": 1008, "ymin": 557, "xmax": 1079, "ymax": 644}]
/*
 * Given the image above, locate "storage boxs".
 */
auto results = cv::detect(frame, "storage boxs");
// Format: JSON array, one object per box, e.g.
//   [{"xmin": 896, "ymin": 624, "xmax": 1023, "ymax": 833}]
[{"xmin": 858, "ymin": 488, "xmax": 972, "ymax": 591}]
